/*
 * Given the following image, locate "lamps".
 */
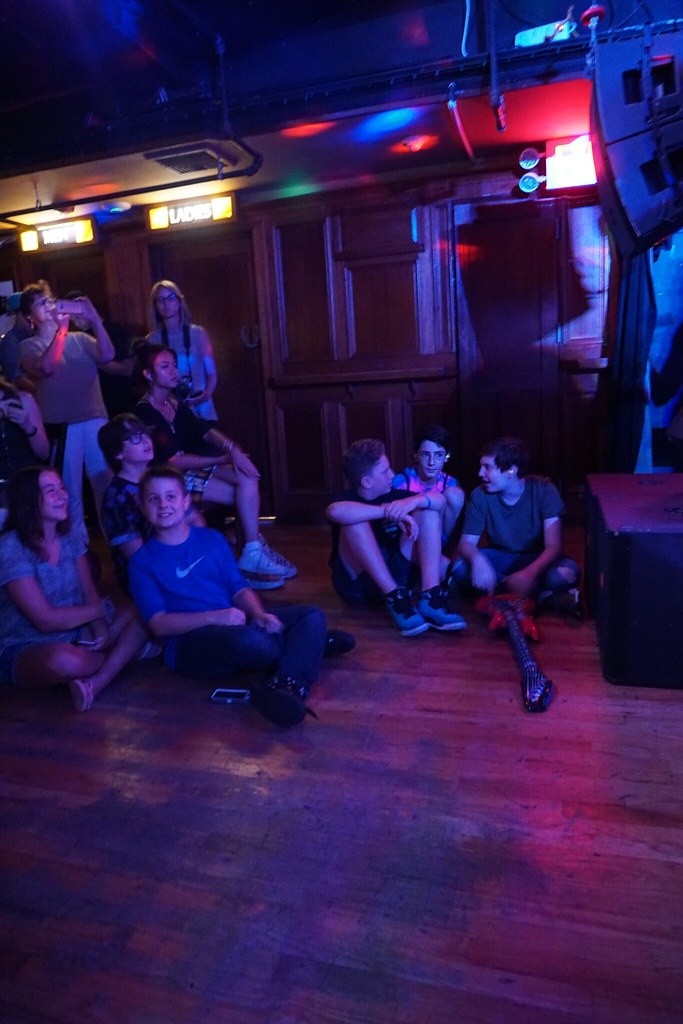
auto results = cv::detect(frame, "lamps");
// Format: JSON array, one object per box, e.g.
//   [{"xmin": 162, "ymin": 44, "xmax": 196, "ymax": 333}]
[{"xmin": 517, "ymin": 134, "xmax": 596, "ymax": 193}]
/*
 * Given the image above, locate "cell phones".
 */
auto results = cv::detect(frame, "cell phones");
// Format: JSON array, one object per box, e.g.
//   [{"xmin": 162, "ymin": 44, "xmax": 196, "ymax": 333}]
[
  {"xmin": 73, "ymin": 639, "xmax": 96, "ymax": 646},
  {"xmin": 208, "ymin": 687, "xmax": 252, "ymax": 704},
  {"xmin": 191, "ymin": 390, "xmax": 203, "ymax": 398},
  {"xmin": 54, "ymin": 298, "xmax": 84, "ymax": 314}
]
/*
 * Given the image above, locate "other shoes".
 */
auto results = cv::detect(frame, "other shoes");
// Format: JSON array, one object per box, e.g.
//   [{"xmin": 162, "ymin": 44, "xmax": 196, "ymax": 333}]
[
  {"xmin": 69, "ymin": 678, "xmax": 95, "ymax": 712},
  {"xmin": 539, "ymin": 586, "xmax": 583, "ymax": 621},
  {"xmin": 135, "ymin": 638, "xmax": 164, "ymax": 661}
]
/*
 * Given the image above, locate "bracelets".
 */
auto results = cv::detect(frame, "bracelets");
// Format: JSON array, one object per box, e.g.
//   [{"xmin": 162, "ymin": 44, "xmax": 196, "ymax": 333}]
[
  {"xmin": 419, "ymin": 493, "xmax": 431, "ymax": 510},
  {"xmin": 223, "ymin": 438, "xmax": 235, "ymax": 454},
  {"xmin": 26, "ymin": 426, "xmax": 38, "ymax": 437},
  {"xmin": 56, "ymin": 331, "xmax": 68, "ymax": 336}
]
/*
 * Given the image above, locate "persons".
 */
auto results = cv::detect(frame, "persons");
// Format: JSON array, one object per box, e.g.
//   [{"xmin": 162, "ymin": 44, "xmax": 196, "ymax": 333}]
[
  {"xmin": 126, "ymin": 468, "xmax": 355, "ymax": 727},
  {"xmin": 0, "ymin": 291, "xmax": 136, "ymax": 535},
  {"xmin": 98, "ymin": 413, "xmax": 205, "ymax": 597},
  {"xmin": 130, "ymin": 339, "xmax": 297, "ymax": 590},
  {"xmin": 326, "ymin": 439, "xmax": 467, "ymax": 637},
  {"xmin": 451, "ymin": 438, "xmax": 579, "ymax": 609},
  {"xmin": 392, "ymin": 423, "xmax": 465, "ymax": 589},
  {"xmin": 0, "ymin": 468, "xmax": 149, "ymax": 711},
  {"xmin": 19, "ymin": 281, "xmax": 116, "ymax": 548},
  {"xmin": 145, "ymin": 281, "xmax": 220, "ymax": 430}
]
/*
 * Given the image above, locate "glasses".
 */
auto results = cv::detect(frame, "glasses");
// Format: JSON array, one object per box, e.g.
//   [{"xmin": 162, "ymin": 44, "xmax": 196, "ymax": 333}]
[
  {"xmin": 30, "ymin": 296, "xmax": 58, "ymax": 310},
  {"xmin": 120, "ymin": 431, "xmax": 148, "ymax": 445},
  {"xmin": 156, "ymin": 292, "xmax": 179, "ymax": 303}
]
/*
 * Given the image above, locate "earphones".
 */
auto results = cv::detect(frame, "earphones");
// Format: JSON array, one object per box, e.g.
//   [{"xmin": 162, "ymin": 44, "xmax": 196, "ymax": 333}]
[
  {"xmin": 507, "ymin": 469, "xmax": 513, "ymax": 475},
  {"xmin": 445, "ymin": 455, "xmax": 450, "ymax": 458}
]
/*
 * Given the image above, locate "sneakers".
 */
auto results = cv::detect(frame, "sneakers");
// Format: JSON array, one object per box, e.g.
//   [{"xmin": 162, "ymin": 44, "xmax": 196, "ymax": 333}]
[
  {"xmin": 417, "ymin": 583, "xmax": 468, "ymax": 630},
  {"xmin": 383, "ymin": 584, "xmax": 430, "ymax": 637},
  {"xmin": 245, "ymin": 675, "xmax": 319, "ymax": 727},
  {"xmin": 324, "ymin": 629, "xmax": 359, "ymax": 656},
  {"xmin": 236, "ymin": 537, "xmax": 298, "ymax": 590}
]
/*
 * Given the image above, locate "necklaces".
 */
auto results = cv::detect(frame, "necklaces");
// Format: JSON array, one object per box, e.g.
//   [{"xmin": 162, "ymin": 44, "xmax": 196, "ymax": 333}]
[{"xmin": 146, "ymin": 392, "xmax": 168, "ymax": 405}]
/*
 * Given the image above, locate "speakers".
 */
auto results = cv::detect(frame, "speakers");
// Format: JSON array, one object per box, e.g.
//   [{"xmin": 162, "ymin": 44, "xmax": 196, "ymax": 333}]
[
  {"xmin": 578, "ymin": 469, "xmax": 683, "ymax": 691},
  {"xmin": 588, "ymin": 30, "xmax": 683, "ymax": 264}
]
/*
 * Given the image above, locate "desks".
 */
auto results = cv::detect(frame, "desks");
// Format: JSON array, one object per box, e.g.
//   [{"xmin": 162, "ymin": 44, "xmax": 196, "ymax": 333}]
[{"xmin": 584, "ymin": 469, "xmax": 683, "ymax": 688}]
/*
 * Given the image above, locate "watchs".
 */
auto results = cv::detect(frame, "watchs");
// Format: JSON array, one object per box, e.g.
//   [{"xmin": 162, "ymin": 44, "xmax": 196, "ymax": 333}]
[{"xmin": 101, "ymin": 598, "xmax": 112, "ymax": 616}]
